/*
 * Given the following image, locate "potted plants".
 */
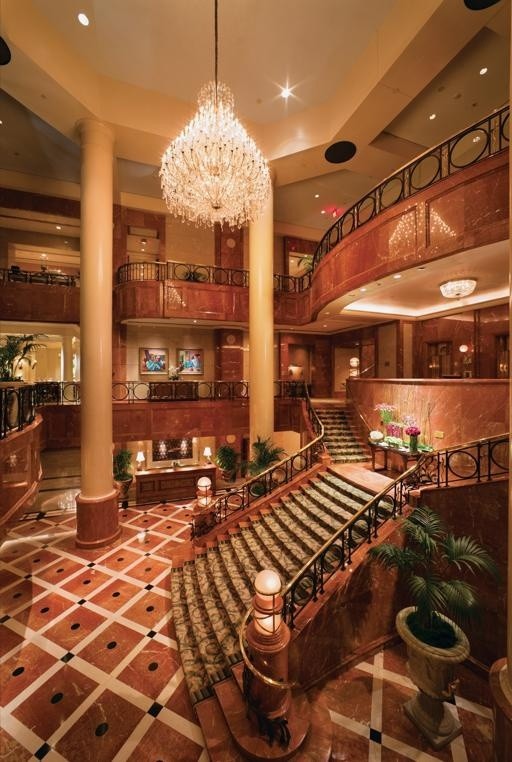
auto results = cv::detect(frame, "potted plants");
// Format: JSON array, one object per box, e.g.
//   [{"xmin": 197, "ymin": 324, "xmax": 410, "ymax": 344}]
[
  {"xmin": 244, "ymin": 435, "xmax": 292, "ymax": 498},
  {"xmin": 371, "ymin": 502, "xmax": 507, "ymax": 750},
  {"xmin": 214, "ymin": 446, "xmax": 237, "ymax": 483},
  {"xmin": 112, "ymin": 449, "xmax": 133, "ymax": 500}
]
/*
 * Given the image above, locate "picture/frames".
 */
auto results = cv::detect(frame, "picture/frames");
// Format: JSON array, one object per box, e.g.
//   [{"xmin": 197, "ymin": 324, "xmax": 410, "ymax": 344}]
[
  {"xmin": 176, "ymin": 347, "xmax": 204, "ymax": 375},
  {"xmin": 138, "ymin": 347, "xmax": 170, "ymax": 375}
]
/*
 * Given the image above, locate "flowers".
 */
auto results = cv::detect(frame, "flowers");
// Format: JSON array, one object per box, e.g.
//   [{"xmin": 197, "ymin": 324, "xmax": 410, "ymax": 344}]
[{"xmin": 370, "ymin": 401, "xmax": 422, "ymax": 454}]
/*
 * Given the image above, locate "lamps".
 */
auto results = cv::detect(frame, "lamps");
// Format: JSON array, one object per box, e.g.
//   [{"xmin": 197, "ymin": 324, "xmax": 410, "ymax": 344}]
[
  {"xmin": 195, "ymin": 476, "xmax": 213, "ymax": 510},
  {"xmin": 203, "ymin": 447, "xmax": 212, "ymax": 461},
  {"xmin": 252, "ymin": 570, "xmax": 284, "ymax": 645},
  {"xmin": 135, "ymin": 451, "xmax": 145, "ymax": 472},
  {"xmin": 348, "ymin": 357, "xmax": 361, "ymax": 379},
  {"xmin": 158, "ymin": 0, "xmax": 271, "ymax": 236},
  {"xmin": 440, "ymin": 277, "xmax": 477, "ymax": 298}
]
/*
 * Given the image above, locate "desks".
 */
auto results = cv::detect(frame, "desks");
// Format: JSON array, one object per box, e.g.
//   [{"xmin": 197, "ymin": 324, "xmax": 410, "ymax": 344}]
[{"xmin": 366, "ymin": 441, "xmax": 419, "ymax": 473}]
